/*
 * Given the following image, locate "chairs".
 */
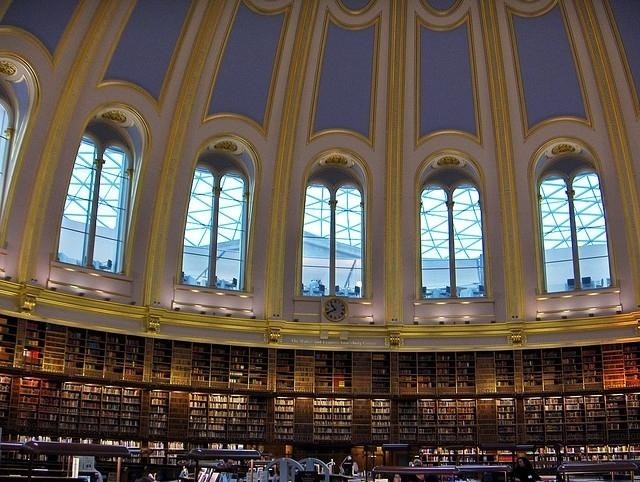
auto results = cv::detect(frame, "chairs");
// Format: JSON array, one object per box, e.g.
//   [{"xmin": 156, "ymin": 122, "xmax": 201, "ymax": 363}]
[
  {"xmin": 422, "ymin": 282, "xmax": 484, "ymax": 299},
  {"xmin": 56, "ymin": 252, "xmax": 117, "ymax": 276},
  {"xmin": 301, "ymin": 278, "xmax": 362, "ymax": 299},
  {"xmin": 548, "ymin": 273, "xmax": 610, "ymax": 293},
  {"xmin": 180, "ymin": 269, "xmax": 241, "ymax": 290}
]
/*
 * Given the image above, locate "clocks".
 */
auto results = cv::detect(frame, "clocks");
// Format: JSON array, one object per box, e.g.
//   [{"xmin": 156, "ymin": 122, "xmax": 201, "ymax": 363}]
[{"xmin": 322, "ymin": 296, "xmax": 350, "ymax": 322}]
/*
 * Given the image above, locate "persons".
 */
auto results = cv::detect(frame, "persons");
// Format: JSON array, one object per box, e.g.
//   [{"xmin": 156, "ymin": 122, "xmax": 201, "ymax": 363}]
[
  {"xmin": 514, "ymin": 457, "xmax": 541, "ymax": 482},
  {"xmin": 391, "ymin": 474, "xmax": 402, "ymax": 482},
  {"xmin": 416, "ymin": 474, "xmax": 439, "ymax": 482}
]
